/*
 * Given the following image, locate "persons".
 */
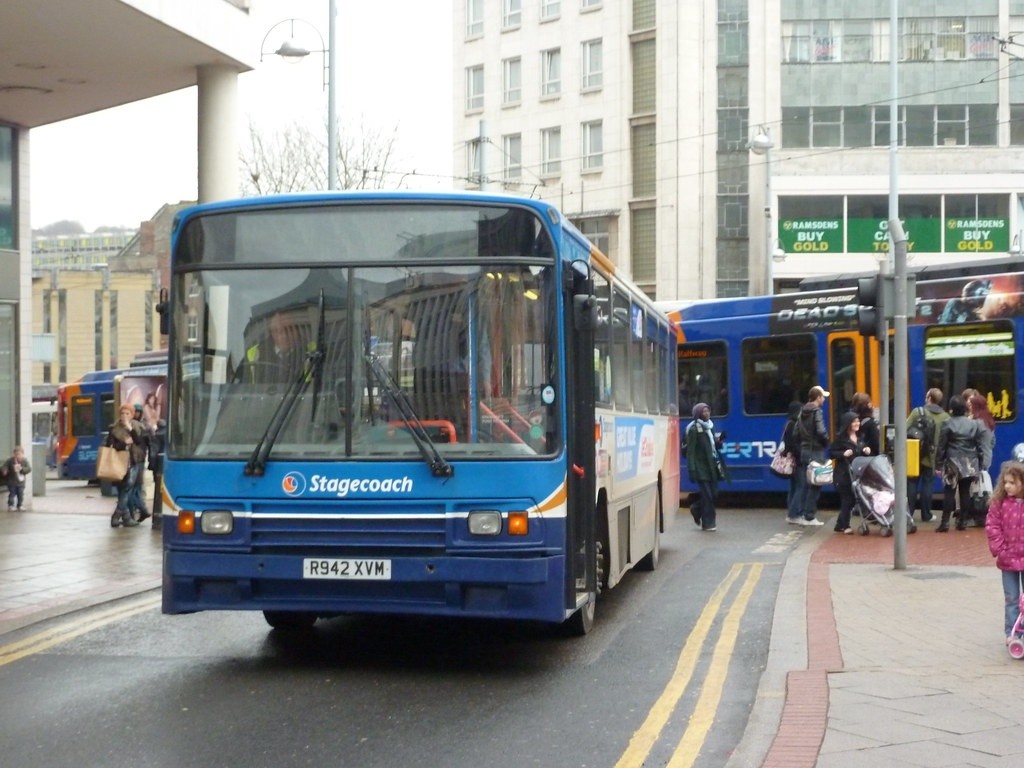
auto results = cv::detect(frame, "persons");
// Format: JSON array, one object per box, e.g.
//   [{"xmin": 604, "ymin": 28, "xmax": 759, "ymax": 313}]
[
  {"xmin": 686, "ymin": 403, "xmax": 728, "ymax": 531},
  {"xmin": 782, "ymin": 385, "xmax": 996, "ymax": 537},
  {"xmin": 143, "ymin": 394, "xmax": 161, "ymax": 431},
  {"xmin": 986, "ymin": 460, "xmax": 1024, "ymax": 645},
  {"xmin": 230, "ymin": 309, "xmax": 316, "ymax": 383},
  {"xmin": 105, "ymin": 402, "xmax": 166, "ymax": 527},
  {"xmin": 1, "ymin": 446, "xmax": 32, "ymax": 511}
]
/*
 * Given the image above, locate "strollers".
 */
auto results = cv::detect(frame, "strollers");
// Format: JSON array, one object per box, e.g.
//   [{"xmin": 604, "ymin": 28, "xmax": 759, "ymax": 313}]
[
  {"xmin": 843, "ymin": 446, "xmax": 917, "ymax": 538},
  {"xmin": 1006, "ymin": 594, "xmax": 1024, "ymax": 660}
]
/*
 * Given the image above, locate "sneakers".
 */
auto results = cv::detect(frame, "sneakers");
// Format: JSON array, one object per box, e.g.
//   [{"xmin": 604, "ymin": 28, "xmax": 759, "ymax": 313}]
[{"xmin": 785, "ymin": 513, "xmax": 824, "ymax": 526}]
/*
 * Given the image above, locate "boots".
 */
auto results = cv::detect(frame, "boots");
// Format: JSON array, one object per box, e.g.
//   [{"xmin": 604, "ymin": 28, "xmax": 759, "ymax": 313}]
[
  {"xmin": 955, "ymin": 507, "xmax": 968, "ymax": 531},
  {"xmin": 112, "ymin": 506, "xmax": 121, "ymax": 527},
  {"xmin": 122, "ymin": 509, "xmax": 140, "ymax": 527},
  {"xmin": 935, "ymin": 509, "xmax": 951, "ymax": 533}
]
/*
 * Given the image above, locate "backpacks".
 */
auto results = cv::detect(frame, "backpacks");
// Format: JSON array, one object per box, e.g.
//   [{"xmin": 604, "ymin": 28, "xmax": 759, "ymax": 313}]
[{"xmin": 907, "ymin": 407, "xmax": 936, "ymax": 460}]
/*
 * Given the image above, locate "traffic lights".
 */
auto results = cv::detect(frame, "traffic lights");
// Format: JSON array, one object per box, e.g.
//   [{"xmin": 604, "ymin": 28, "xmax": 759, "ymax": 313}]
[{"xmin": 856, "ymin": 274, "xmax": 886, "ymax": 343}]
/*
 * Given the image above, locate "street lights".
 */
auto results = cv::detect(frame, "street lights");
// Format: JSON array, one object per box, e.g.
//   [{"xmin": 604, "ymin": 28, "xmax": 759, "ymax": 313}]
[
  {"xmin": 745, "ymin": 125, "xmax": 774, "ymax": 297},
  {"xmin": 256, "ymin": 1, "xmax": 341, "ymax": 190}
]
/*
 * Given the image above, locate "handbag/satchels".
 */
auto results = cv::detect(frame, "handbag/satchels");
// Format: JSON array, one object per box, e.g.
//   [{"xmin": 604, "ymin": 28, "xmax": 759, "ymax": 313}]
[
  {"xmin": 969, "ymin": 470, "xmax": 993, "ymax": 506},
  {"xmin": 769, "ymin": 450, "xmax": 795, "ymax": 475},
  {"xmin": 96, "ymin": 443, "xmax": 130, "ymax": 480},
  {"xmin": 806, "ymin": 459, "xmax": 834, "ymax": 486}
]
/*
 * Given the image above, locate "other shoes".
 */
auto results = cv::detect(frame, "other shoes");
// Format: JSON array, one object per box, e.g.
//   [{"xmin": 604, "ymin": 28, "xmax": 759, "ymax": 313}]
[
  {"xmin": 137, "ymin": 511, "xmax": 151, "ymax": 522},
  {"xmin": 834, "ymin": 526, "xmax": 853, "ymax": 534},
  {"xmin": 928, "ymin": 515, "xmax": 937, "ymax": 521},
  {"xmin": 1005, "ymin": 635, "xmax": 1019, "ymax": 646},
  {"xmin": 18, "ymin": 506, "xmax": 26, "ymax": 511},
  {"xmin": 691, "ymin": 505, "xmax": 716, "ymax": 532},
  {"xmin": 8, "ymin": 505, "xmax": 17, "ymax": 512}
]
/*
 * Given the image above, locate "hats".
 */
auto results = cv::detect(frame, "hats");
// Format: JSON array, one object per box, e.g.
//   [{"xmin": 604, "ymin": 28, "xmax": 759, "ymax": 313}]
[
  {"xmin": 135, "ymin": 404, "xmax": 143, "ymax": 421},
  {"xmin": 692, "ymin": 402, "xmax": 710, "ymax": 420},
  {"xmin": 808, "ymin": 386, "xmax": 830, "ymax": 402},
  {"xmin": 852, "ymin": 392, "xmax": 869, "ymax": 408},
  {"xmin": 120, "ymin": 404, "xmax": 135, "ymax": 416}
]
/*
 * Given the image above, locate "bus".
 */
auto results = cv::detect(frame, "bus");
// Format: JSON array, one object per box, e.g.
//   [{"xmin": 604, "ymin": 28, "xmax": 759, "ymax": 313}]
[
  {"xmin": 666, "ymin": 269, "xmax": 1024, "ymax": 497},
  {"xmin": 49, "ymin": 360, "xmax": 199, "ymax": 481},
  {"xmin": 148, "ymin": 187, "xmax": 679, "ymax": 637}
]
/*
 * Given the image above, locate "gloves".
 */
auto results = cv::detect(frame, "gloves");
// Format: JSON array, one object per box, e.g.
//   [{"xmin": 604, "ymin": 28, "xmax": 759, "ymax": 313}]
[
  {"xmin": 719, "ymin": 432, "xmax": 726, "ymax": 440},
  {"xmin": 688, "ymin": 470, "xmax": 696, "ymax": 483}
]
